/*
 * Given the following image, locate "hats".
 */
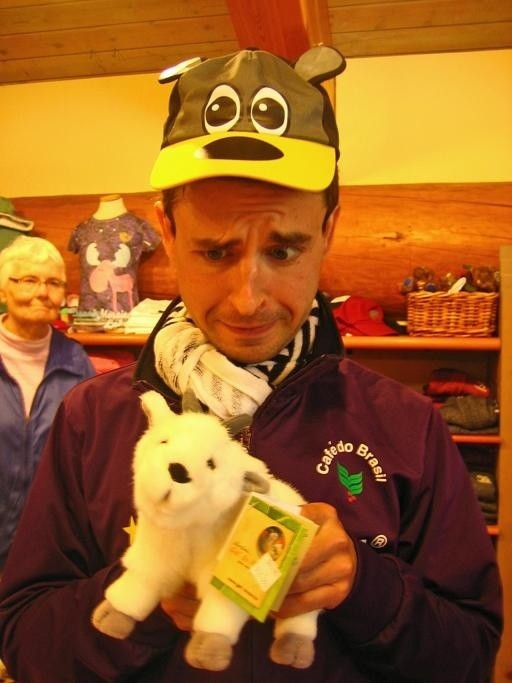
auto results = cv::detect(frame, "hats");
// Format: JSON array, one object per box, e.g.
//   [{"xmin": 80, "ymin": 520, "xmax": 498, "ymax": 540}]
[{"xmin": 148, "ymin": 45, "xmax": 346, "ymax": 194}]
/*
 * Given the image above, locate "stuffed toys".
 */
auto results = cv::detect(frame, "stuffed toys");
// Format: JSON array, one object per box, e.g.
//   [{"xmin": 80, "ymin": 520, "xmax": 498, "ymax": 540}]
[
  {"xmin": 399, "ymin": 261, "xmax": 502, "ymax": 295},
  {"xmin": 91, "ymin": 388, "xmax": 323, "ymax": 673}
]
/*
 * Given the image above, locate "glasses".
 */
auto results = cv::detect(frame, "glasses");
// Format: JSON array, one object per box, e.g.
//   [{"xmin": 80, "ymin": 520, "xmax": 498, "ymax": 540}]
[{"xmin": 9, "ymin": 275, "xmax": 68, "ymax": 292}]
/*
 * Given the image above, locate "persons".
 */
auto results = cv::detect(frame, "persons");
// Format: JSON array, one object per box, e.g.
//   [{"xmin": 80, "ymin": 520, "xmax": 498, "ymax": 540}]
[
  {"xmin": 67, "ymin": 192, "xmax": 161, "ymax": 314},
  {"xmin": 1, "ymin": 231, "xmax": 95, "ymax": 568},
  {"xmin": 3, "ymin": 37, "xmax": 508, "ymax": 682}
]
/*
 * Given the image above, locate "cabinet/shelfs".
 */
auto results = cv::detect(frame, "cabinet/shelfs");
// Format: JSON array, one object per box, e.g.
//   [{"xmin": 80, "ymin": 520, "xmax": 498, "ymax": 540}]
[{"xmin": 64, "ymin": 329, "xmax": 500, "ymax": 536}]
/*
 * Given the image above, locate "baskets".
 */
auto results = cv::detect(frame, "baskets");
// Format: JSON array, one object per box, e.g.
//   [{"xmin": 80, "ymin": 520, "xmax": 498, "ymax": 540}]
[{"xmin": 406, "ymin": 291, "xmax": 501, "ymax": 339}]
